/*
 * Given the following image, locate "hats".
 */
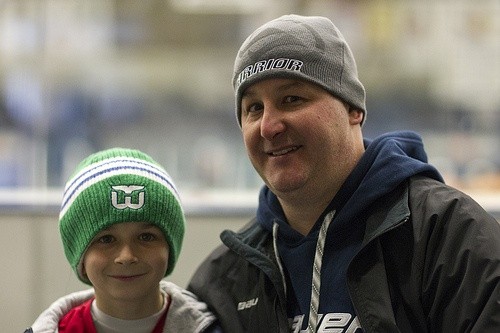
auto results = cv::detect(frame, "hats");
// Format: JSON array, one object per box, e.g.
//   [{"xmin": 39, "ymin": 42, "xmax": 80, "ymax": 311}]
[
  {"xmin": 58, "ymin": 148, "xmax": 185, "ymax": 287},
  {"xmin": 233, "ymin": 13, "xmax": 367, "ymax": 133}
]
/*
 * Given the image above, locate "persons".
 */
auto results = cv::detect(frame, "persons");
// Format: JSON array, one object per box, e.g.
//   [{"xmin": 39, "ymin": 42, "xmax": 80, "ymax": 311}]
[
  {"xmin": 23, "ymin": 146, "xmax": 222, "ymax": 333},
  {"xmin": 184, "ymin": 12, "xmax": 500, "ymax": 333}
]
